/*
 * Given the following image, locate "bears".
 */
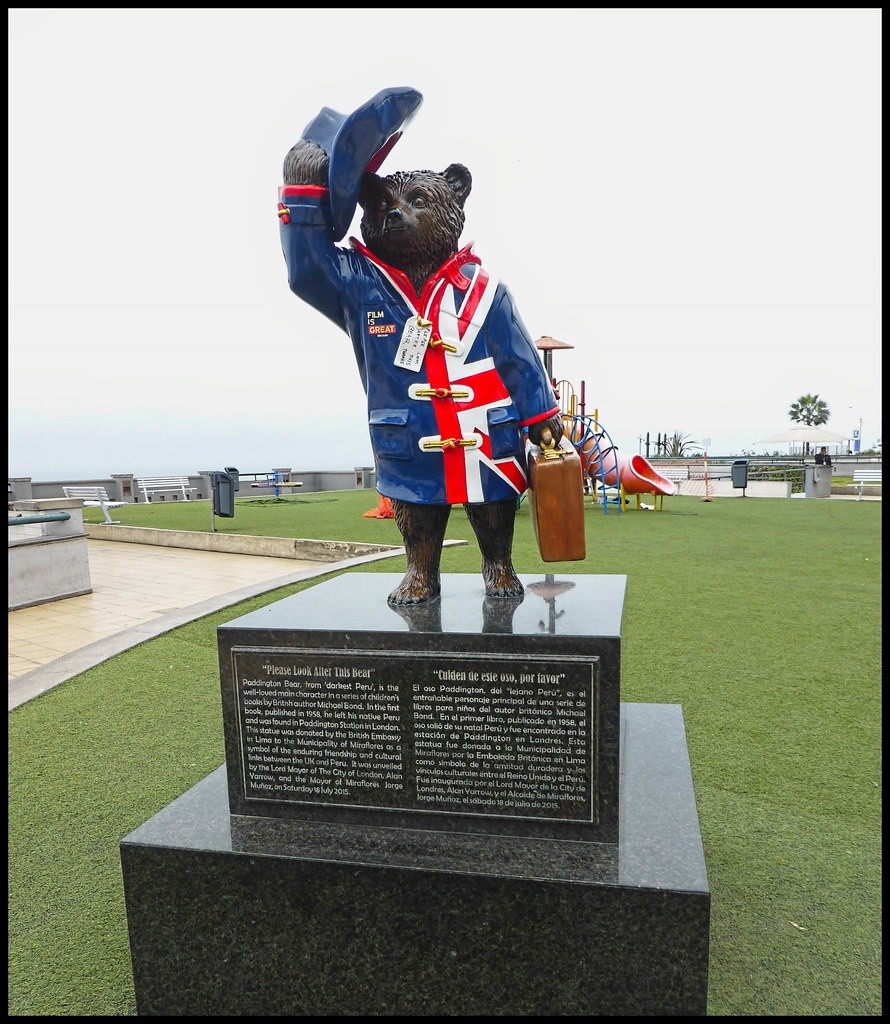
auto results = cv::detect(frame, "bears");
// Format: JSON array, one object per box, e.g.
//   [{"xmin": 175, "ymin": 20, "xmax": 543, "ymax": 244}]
[{"xmin": 274, "ymin": 86, "xmax": 568, "ymax": 608}]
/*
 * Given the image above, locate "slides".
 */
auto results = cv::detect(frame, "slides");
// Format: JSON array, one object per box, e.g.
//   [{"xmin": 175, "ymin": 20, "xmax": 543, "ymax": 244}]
[{"xmin": 565, "ymin": 419, "xmax": 678, "ymax": 497}]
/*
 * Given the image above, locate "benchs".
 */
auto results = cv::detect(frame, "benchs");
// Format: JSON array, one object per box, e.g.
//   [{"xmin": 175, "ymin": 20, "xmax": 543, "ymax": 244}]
[
  {"xmin": 657, "ymin": 469, "xmax": 689, "ymax": 495},
  {"xmin": 61, "ymin": 487, "xmax": 128, "ymax": 525},
  {"xmin": 848, "ymin": 470, "xmax": 882, "ymax": 501},
  {"xmin": 137, "ymin": 476, "xmax": 198, "ymax": 504}
]
